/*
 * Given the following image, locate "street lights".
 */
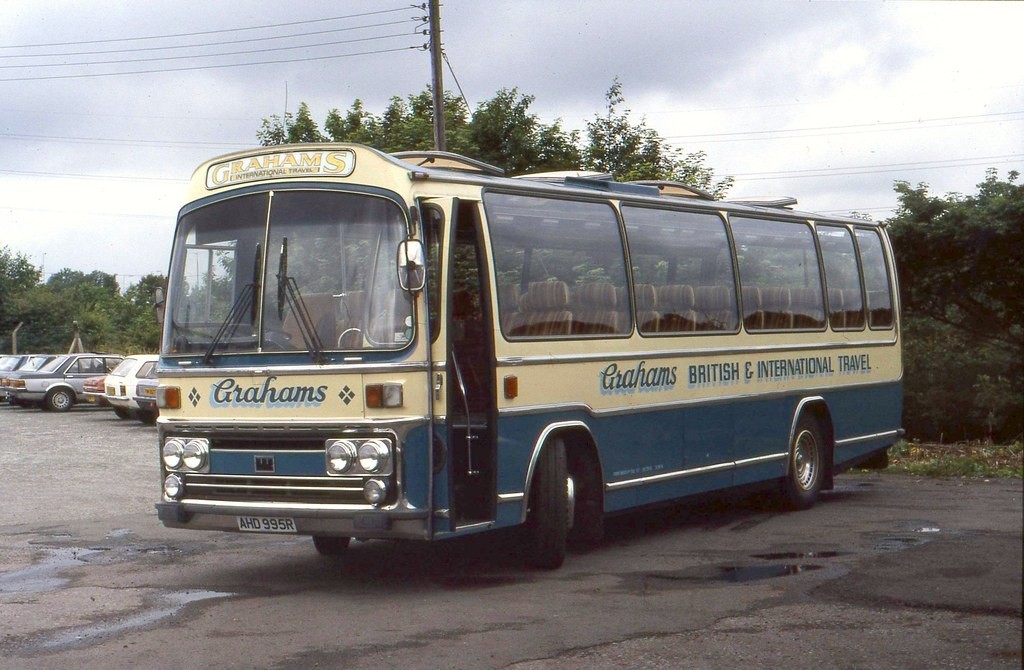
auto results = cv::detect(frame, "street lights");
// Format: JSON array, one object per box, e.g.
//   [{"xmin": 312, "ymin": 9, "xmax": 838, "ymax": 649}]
[
  {"xmin": 43, "ymin": 253, "xmax": 47, "ymax": 285},
  {"xmin": 193, "ymin": 252, "xmax": 199, "ymax": 292}
]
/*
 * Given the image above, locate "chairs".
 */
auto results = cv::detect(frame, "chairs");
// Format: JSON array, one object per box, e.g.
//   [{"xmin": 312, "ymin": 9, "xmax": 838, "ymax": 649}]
[
  {"xmin": 497, "ymin": 281, "xmax": 893, "ymax": 336},
  {"xmin": 282, "ymin": 291, "xmax": 379, "ymax": 351}
]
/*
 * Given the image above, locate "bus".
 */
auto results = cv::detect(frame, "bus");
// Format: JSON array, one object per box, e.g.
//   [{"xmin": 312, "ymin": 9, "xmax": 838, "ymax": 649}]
[{"xmin": 153, "ymin": 142, "xmax": 905, "ymax": 569}]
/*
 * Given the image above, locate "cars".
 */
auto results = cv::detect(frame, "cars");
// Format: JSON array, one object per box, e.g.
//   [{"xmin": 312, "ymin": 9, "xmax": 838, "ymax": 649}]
[
  {"xmin": 103, "ymin": 355, "xmax": 160, "ymax": 424},
  {"xmin": 0, "ymin": 353, "xmax": 125, "ymax": 412}
]
